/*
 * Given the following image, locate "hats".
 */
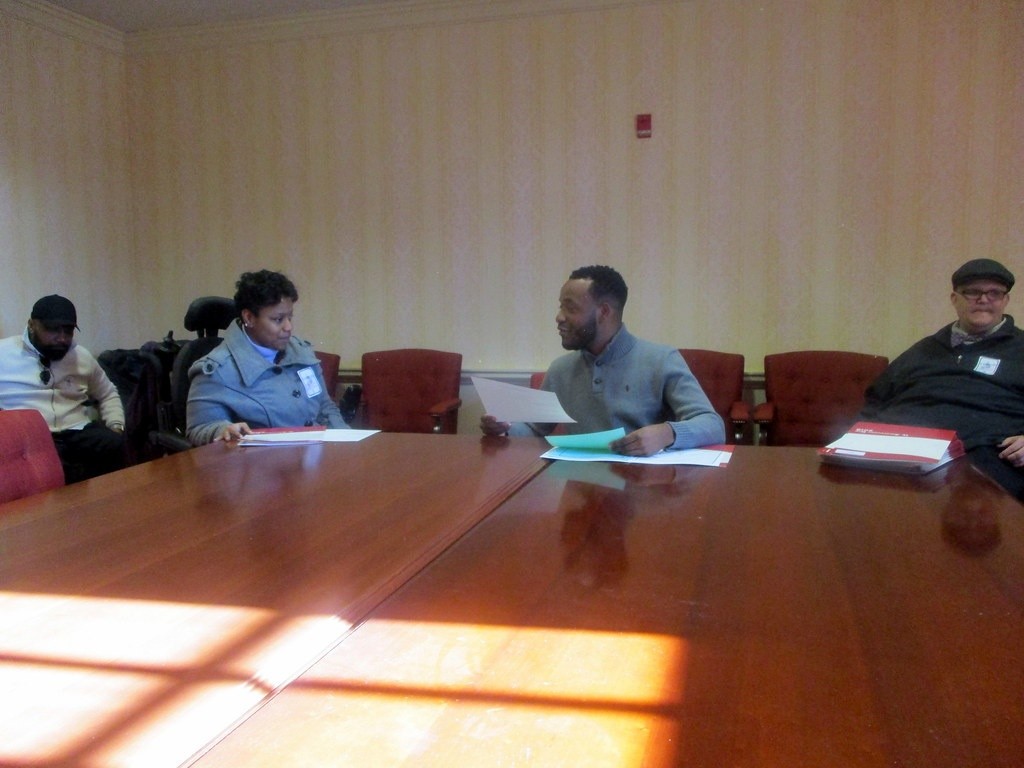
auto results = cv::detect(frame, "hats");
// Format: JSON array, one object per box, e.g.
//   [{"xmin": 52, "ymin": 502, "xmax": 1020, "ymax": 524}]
[
  {"xmin": 951, "ymin": 258, "xmax": 1015, "ymax": 293},
  {"xmin": 31, "ymin": 294, "xmax": 80, "ymax": 332}
]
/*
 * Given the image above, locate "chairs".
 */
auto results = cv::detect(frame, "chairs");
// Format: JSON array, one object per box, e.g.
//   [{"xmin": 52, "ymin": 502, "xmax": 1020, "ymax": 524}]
[
  {"xmin": 530, "ymin": 371, "xmax": 564, "ymax": 435},
  {"xmin": 170, "ymin": 296, "xmax": 238, "ymax": 427},
  {"xmin": 752, "ymin": 350, "xmax": 889, "ymax": 447},
  {"xmin": 314, "ymin": 351, "xmax": 342, "ymax": 400},
  {"xmin": 0, "ymin": 408, "xmax": 66, "ymax": 504},
  {"xmin": 350, "ymin": 348, "xmax": 463, "ymax": 434},
  {"xmin": 677, "ymin": 348, "xmax": 752, "ymax": 445}
]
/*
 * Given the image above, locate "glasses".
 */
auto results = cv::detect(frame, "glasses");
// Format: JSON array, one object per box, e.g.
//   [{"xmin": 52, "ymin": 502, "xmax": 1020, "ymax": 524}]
[
  {"xmin": 39, "ymin": 355, "xmax": 52, "ymax": 385},
  {"xmin": 953, "ymin": 287, "xmax": 1010, "ymax": 301}
]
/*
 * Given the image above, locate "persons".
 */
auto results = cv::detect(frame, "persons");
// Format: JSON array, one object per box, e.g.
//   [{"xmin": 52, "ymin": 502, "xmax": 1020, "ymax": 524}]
[
  {"xmin": 939, "ymin": 465, "xmax": 1003, "ymax": 557},
  {"xmin": 479, "ymin": 265, "xmax": 725, "ymax": 457},
  {"xmin": 0, "ymin": 294, "xmax": 126, "ymax": 485},
  {"xmin": 186, "ymin": 269, "xmax": 352, "ymax": 447},
  {"xmin": 860, "ymin": 258, "xmax": 1024, "ymax": 507}
]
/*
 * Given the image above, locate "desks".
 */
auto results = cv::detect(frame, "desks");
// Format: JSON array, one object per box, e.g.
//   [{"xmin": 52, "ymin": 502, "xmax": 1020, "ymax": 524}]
[
  {"xmin": 190, "ymin": 447, "xmax": 1024, "ymax": 768},
  {"xmin": 0, "ymin": 429, "xmax": 553, "ymax": 768}
]
